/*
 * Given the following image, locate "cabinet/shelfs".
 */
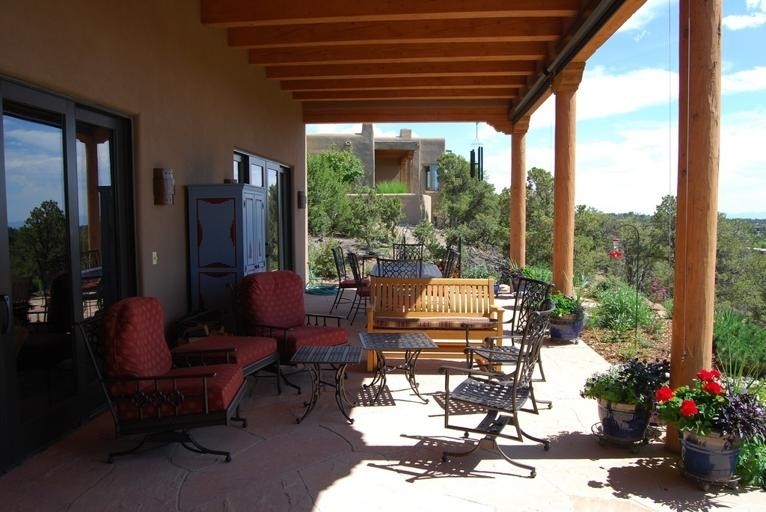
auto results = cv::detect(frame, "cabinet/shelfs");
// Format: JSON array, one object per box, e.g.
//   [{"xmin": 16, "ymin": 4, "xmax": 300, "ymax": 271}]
[{"xmin": 183, "ymin": 182, "xmax": 270, "ymax": 335}]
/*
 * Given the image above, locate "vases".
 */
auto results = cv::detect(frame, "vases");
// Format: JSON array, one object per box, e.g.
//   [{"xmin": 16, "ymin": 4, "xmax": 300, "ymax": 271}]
[{"xmin": 678, "ymin": 421, "xmax": 742, "ymax": 481}]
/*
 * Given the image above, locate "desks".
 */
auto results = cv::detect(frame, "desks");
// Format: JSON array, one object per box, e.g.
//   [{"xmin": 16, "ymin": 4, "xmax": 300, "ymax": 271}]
[{"xmin": 171, "ymin": 328, "xmax": 281, "ymax": 421}]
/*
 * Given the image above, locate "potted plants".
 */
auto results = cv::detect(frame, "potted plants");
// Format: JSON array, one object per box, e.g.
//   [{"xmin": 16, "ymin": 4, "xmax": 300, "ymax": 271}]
[
  {"xmin": 584, "ymin": 358, "xmax": 671, "ymax": 447},
  {"xmin": 545, "ymin": 291, "xmax": 584, "ymax": 343}
]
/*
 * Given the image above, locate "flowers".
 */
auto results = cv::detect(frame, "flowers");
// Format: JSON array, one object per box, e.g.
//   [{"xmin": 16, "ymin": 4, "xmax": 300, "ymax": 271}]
[{"xmin": 655, "ymin": 363, "xmax": 766, "ymax": 441}]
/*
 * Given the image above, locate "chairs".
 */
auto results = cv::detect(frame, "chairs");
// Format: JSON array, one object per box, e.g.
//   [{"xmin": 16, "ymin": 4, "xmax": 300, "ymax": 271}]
[
  {"xmin": 433, "ymin": 275, "xmax": 560, "ymax": 476},
  {"xmin": 239, "ymin": 268, "xmax": 349, "ymax": 394},
  {"xmin": 328, "ymin": 245, "xmax": 460, "ymax": 325},
  {"xmin": 71, "ymin": 294, "xmax": 245, "ymax": 467}
]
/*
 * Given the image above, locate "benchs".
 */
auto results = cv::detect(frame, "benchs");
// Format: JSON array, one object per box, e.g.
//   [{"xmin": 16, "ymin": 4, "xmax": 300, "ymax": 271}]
[{"xmin": 364, "ymin": 274, "xmax": 504, "ymax": 378}]
